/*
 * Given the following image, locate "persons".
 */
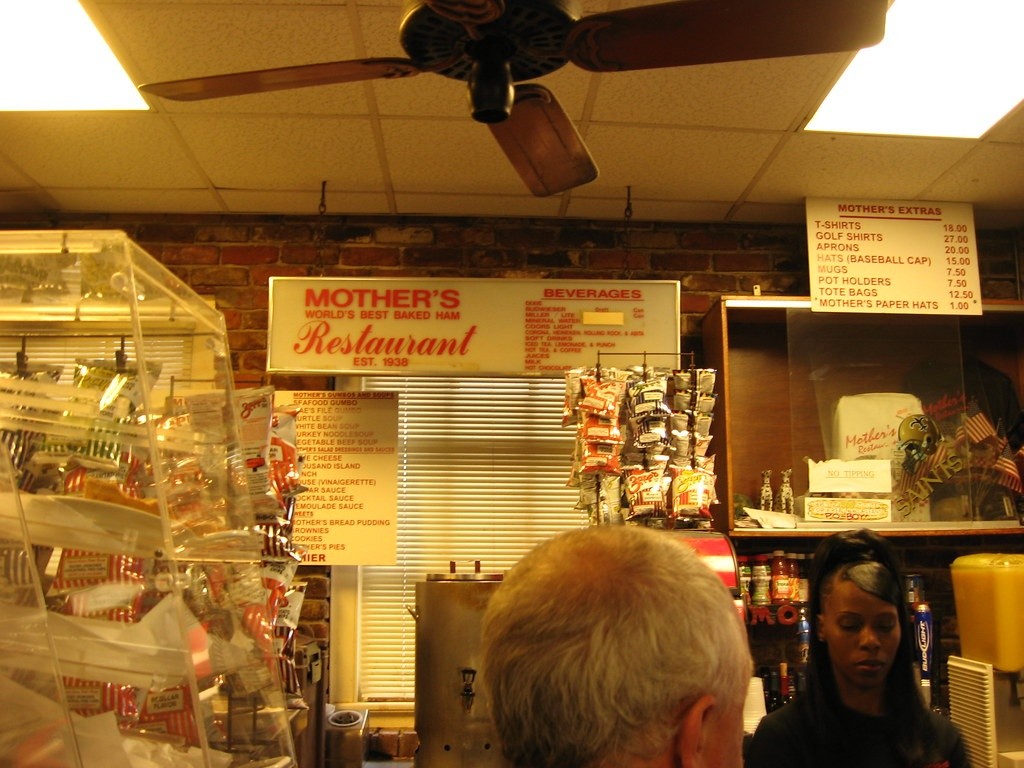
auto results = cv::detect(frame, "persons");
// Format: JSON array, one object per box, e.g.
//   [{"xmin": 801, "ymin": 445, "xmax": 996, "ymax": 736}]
[
  {"xmin": 741, "ymin": 526, "xmax": 971, "ymax": 768},
  {"xmin": 477, "ymin": 524, "xmax": 760, "ymax": 768}
]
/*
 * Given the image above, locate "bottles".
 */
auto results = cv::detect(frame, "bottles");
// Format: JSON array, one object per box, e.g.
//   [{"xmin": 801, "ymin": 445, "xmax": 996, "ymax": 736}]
[
  {"xmin": 751, "ymin": 555, "xmax": 772, "ymax": 606},
  {"xmin": 758, "ymin": 661, "xmax": 798, "ymax": 712},
  {"xmin": 771, "ymin": 550, "xmax": 789, "ymax": 603},
  {"xmin": 785, "ymin": 553, "xmax": 798, "ymax": 602},
  {"xmin": 738, "ymin": 556, "xmax": 751, "ymax": 605},
  {"xmin": 797, "ymin": 554, "xmax": 810, "ymax": 603}
]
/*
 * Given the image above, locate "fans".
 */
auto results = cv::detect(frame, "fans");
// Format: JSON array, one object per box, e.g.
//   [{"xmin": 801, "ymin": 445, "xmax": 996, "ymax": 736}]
[{"xmin": 137, "ymin": 0, "xmax": 885, "ymax": 198}]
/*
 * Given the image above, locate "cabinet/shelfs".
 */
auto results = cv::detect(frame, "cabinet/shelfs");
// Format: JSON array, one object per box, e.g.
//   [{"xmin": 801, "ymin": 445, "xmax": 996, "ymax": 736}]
[
  {"xmin": 0, "ymin": 228, "xmax": 302, "ymax": 768},
  {"xmin": 697, "ymin": 294, "xmax": 1024, "ymax": 537}
]
